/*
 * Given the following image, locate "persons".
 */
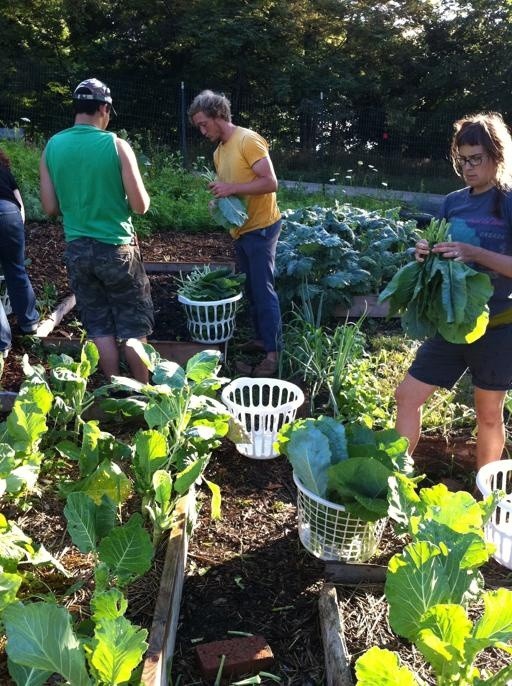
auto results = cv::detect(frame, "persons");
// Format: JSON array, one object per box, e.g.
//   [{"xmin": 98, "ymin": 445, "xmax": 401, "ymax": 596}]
[
  {"xmin": 44, "ymin": 78, "xmax": 156, "ymax": 394},
  {"xmin": 393, "ymin": 113, "xmax": 512, "ymax": 499},
  {"xmin": 186, "ymin": 89, "xmax": 284, "ymax": 377},
  {"xmin": 0, "ymin": 150, "xmax": 41, "ymax": 362}
]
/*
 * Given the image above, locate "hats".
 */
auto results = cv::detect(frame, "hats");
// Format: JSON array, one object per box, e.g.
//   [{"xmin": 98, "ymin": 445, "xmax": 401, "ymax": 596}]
[{"xmin": 72, "ymin": 77, "xmax": 118, "ymax": 116}]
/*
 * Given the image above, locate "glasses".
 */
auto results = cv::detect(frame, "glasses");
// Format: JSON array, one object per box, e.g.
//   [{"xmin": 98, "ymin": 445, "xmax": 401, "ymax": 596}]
[{"xmin": 456, "ymin": 155, "xmax": 486, "ymax": 165}]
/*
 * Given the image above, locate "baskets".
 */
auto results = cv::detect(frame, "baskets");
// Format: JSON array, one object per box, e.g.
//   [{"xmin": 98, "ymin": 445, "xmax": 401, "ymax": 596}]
[
  {"xmin": 475, "ymin": 459, "xmax": 512, "ymax": 571},
  {"xmin": 176, "ymin": 290, "xmax": 243, "ymax": 344},
  {"xmin": 292, "ymin": 467, "xmax": 388, "ymax": 562},
  {"xmin": 222, "ymin": 378, "xmax": 304, "ymax": 459}
]
